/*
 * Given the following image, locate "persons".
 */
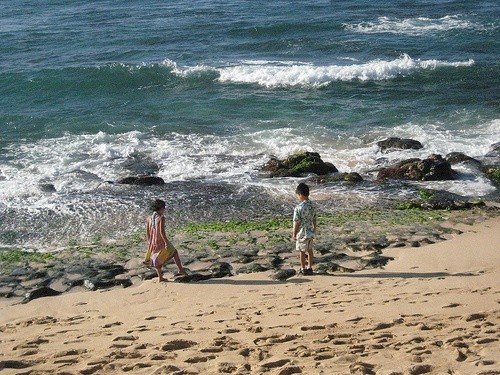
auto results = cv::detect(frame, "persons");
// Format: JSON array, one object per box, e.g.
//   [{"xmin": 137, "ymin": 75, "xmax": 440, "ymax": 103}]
[
  {"xmin": 293, "ymin": 183, "xmax": 316, "ymax": 274},
  {"xmin": 145, "ymin": 199, "xmax": 188, "ymax": 283}
]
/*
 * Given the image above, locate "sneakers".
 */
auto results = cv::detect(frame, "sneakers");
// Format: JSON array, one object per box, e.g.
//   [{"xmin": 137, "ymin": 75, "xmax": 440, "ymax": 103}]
[
  {"xmin": 296, "ymin": 267, "xmax": 308, "ymax": 275},
  {"xmin": 307, "ymin": 268, "xmax": 313, "ymax": 275}
]
